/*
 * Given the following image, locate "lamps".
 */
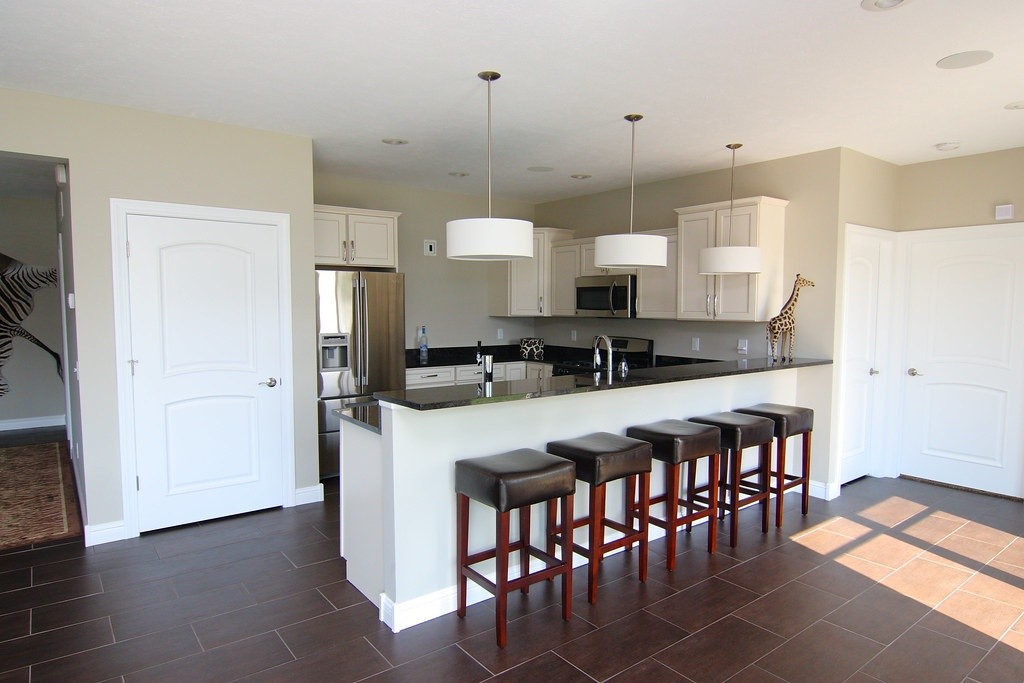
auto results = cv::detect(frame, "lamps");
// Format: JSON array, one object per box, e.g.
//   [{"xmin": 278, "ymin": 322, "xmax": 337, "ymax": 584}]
[
  {"xmin": 445, "ymin": 71, "xmax": 534, "ymax": 263},
  {"xmin": 593, "ymin": 114, "xmax": 667, "ymax": 269},
  {"xmin": 698, "ymin": 144, "xmax": 764, "ymax": 276}
]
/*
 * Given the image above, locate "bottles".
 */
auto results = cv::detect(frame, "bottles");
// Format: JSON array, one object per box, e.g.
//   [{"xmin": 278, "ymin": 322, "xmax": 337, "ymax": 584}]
[{"xmin": 419, "ymin": 325, "xmax": 428, "ymax": 359}]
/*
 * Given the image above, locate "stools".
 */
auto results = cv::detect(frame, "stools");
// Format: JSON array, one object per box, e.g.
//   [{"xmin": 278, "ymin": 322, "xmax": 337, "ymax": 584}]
[
  {"xmin": 625, "ymin": 419, "xmax": 721, "ymax": 574},
  {"xmin": 456, "ymin": 448, "xmax": 576, "ymax": 651},
  {"xmin": 734, "ymin": 402, "xmax": 814, "ymax": 529},
  {"xmin": 687, "ymin": 411, "xmax": 776, "ymax": 550},
  {"xmin": 546, "ymin": 431, "xmax": 652, "ymax": 606}
]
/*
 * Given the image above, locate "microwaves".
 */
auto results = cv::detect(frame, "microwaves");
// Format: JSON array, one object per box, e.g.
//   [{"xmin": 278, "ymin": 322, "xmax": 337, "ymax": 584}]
[{"xmin": 573, "ymin": 273, "xmax": 636, "ymax": 319}]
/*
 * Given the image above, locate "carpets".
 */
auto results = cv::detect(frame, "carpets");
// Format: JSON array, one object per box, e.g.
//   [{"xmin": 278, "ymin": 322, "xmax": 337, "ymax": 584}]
[{"xmin": 0, "ymin": 440, "xmax": 84, "ymax": 550}]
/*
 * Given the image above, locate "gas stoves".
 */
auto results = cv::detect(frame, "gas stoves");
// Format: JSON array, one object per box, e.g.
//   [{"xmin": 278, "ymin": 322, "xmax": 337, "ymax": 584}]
[{"xmin": 552, "ymin": 335, "xmax": 654, "ymax": 375}]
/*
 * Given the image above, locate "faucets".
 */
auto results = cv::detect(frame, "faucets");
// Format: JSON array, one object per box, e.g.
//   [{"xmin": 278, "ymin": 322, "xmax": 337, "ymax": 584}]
[
  {"xmin": 593, "ymin": 333, "xmax": 612, "ymax": 371},
  {"xmin": 593, "ymin": 371, "xmax": 612, "ymax": 387}
]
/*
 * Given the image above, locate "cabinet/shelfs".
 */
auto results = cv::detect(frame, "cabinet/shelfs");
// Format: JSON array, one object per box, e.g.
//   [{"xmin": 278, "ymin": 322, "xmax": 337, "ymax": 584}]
[
  {"xmin": 404, "ymin": 361, "xmax": 554, "ymax": 391},
  {"xmin": 490, "ymin": 195, "xmax": 789, "ymax": 323},
  {"xmin": 313, "ymin": 203, "xmax": 403, "ymax": 268}
]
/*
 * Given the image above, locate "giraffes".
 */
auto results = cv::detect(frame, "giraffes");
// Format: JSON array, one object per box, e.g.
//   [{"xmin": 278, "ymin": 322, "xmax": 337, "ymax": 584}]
[{"xmin": 766, "ymin": 273, "xmax": 815, "ymax": 362}]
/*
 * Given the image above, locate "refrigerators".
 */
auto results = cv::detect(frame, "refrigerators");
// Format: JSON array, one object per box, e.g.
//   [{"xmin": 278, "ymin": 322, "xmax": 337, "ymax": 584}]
[{"xmin": 315, "ymin": 268, "xmax": 407, "ymax": 479}]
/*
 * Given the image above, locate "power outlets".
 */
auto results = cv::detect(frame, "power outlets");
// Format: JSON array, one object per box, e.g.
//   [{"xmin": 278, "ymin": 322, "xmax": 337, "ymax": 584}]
[
  {"xmin": 417, "ymin": 330, "xmax": 427, "ymax": 343},
  {"xmin": 736, "ymin": 339, "xmax": 747, "ymax": 355},
  {"xmin": 497, "ymin": 329, "xmax": 503, "ymax": 340},
  {"xmin": 691, "ymin": 337, "xmax": 699, "ymax": 351},
  {"xmin": 570, "ymin": 330, "xmax": 576, "ymax": 341},
  {"xmin": 767, "ymin": 342, "xmax": 777, "ymax": 356}
]
